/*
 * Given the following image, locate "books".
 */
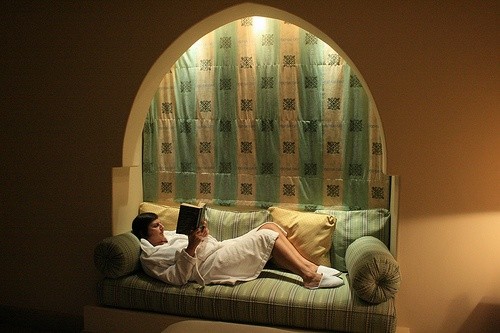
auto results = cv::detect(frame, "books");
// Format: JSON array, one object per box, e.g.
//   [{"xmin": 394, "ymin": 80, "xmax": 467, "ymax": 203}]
[{"xmin": 176, "ymin": 201, "xmax": 206, "ymax": 235}]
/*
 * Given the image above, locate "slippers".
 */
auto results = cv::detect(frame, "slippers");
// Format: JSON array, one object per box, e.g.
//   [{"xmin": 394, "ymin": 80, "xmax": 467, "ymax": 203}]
[
  {"xmin": 308, "ymin": 273, "xmax": 344, "ymax": 290},
  {"xmin": 316, "ymin": 265, "xmax": 342, "ymax": 277}
]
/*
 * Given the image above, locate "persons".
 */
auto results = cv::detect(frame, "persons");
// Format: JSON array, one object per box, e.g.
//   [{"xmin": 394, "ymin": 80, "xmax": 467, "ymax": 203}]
[{"xmin": 132, "ymin": 212, "xmax": 344, "ymax": 290}]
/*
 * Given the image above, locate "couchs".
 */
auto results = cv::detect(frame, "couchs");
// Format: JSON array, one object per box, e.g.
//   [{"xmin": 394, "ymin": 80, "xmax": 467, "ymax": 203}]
[{"xmin": 93, "ymin": 230, "xmax": 402, "ymax": 333}]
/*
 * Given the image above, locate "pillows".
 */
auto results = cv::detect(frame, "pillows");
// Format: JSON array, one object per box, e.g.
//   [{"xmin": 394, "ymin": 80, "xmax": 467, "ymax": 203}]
[
  {"xmin": 205, "ymin": 207, "xmax": 270, "ymax": 242},
  {"xmin": 267, "ymin": 206, "xmax": 337, "ymax": 275},
  {"xmin": 137, "ymin": 202, "xmax": 180, "ymax": 232},
  {"xmin": 314, "ymin": 209, "xmax": 391, "ymax": 273}
]
